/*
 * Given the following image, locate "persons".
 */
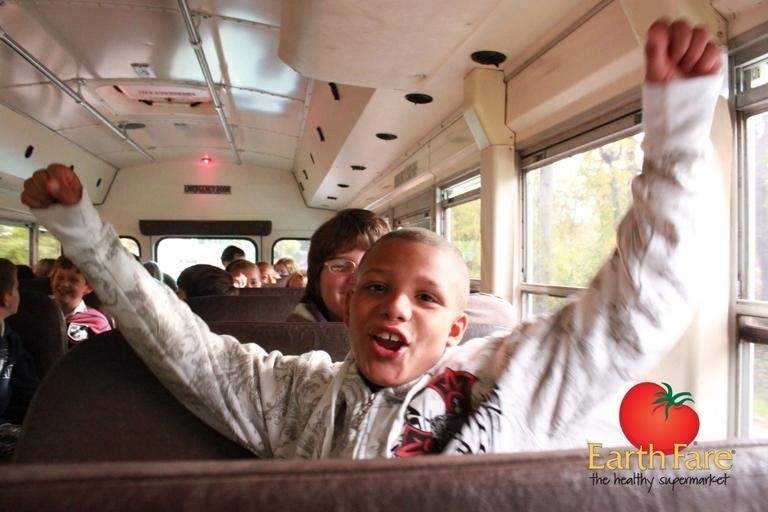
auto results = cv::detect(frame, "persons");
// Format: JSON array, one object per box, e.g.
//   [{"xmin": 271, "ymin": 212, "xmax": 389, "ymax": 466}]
[
  {"xmin": 20, "ymin": 14, "xmax": 724, "ymax": 463},
  {"xmin": 285, "ymin": 205, "xmax": 388, "ymax": 322},
  {"xmin": 1, "ymin": 241, "xmax": 311, "ymax": 450}
]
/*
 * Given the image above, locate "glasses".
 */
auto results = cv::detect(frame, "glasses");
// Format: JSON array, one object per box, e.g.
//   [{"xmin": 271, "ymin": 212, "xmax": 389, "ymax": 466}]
[{"xmin": 323, "ymin": 258, "xmax": 358, "ymax": 276}]
